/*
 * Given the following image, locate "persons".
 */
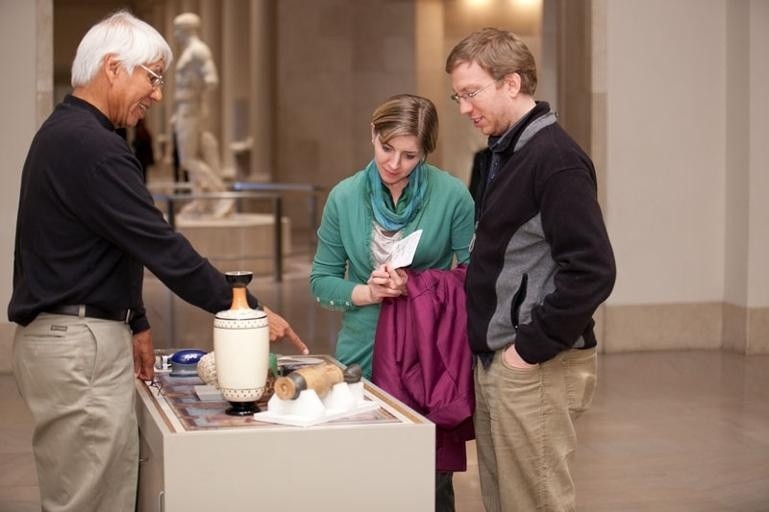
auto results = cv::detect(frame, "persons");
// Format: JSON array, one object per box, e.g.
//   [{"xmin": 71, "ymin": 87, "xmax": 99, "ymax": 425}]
[
  {"xmin": 308, "ymin": 91, "xmax": 480, "ymax": 511},
  {"xmin": 442, "ymin": 26, "xmax": 617, "ymax": 512},
  {"xmin": 131, "ymin": 117, "xmax": 155, "ymax": 189},
  {"xmin": 172, "ymin": 141, "xmax": 190, "ymax": 193},
  {"xmin": 7, "ymin": 6, "xmax": 312, "ymax": 511},
  {"xmin": 174, "ymin": 12, "xmax": 230, "ymax": 217}
]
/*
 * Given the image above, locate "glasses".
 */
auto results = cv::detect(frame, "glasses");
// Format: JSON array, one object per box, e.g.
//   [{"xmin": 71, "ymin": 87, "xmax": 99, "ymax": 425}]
[
  {"xmin": 449, "ymin": 76, "xmax": 501, "ymax": 104},
  {"xmin": 136, "ymin": 62, "xmax": 165, "ymax": 89}
]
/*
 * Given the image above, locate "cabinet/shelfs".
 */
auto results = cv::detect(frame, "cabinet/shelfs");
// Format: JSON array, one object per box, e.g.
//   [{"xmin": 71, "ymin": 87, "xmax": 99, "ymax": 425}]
[{"xmin": 134, "ymin": 348, "xmax": 436, "ymax": 512}]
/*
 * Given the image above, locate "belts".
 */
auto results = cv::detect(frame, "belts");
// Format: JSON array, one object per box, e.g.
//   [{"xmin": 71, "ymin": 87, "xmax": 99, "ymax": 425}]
[{"xmin": 44, "ymin": 301, "xmax": 137, "ymax": 324}]
